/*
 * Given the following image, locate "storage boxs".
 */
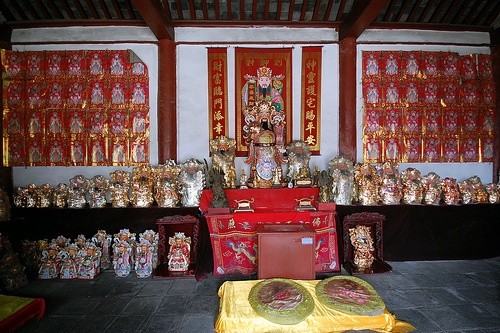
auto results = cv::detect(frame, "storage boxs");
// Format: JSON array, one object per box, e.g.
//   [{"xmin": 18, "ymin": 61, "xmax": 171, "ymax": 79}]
[{"xmin": 257, "ymin": 224, "xmax": 317, "ymax": 280}]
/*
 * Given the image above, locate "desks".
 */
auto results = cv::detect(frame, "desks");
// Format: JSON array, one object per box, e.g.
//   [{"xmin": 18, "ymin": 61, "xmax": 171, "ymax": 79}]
[{"xmin": 204, "ymin": 209, "xmax": 342, "ymax": 277}]
